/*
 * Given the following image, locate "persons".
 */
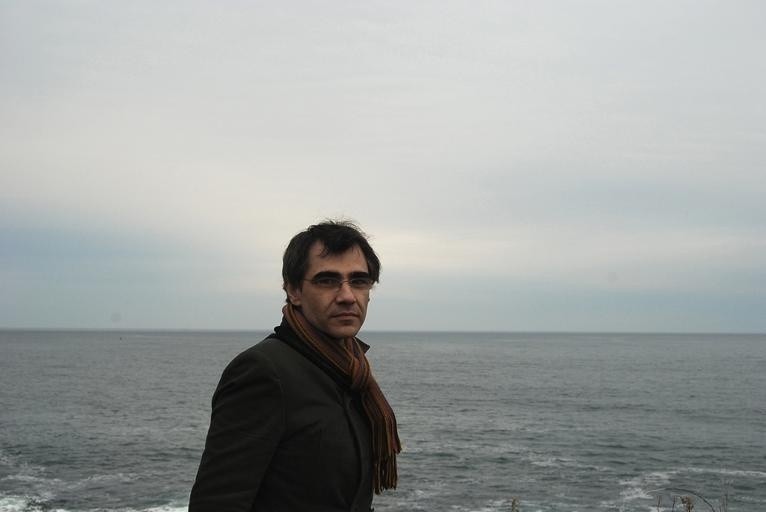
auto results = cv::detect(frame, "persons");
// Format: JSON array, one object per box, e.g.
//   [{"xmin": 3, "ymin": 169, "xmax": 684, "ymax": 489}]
[{"xmin": 188, "ymin": 219, "xmax": 401, "ymax": 512}]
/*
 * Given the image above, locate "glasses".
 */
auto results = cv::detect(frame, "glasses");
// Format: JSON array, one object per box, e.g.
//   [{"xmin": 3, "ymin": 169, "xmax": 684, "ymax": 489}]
[{"xmin": 300, "ymin": 274, "xmax": 374, "ymax": 293}]
